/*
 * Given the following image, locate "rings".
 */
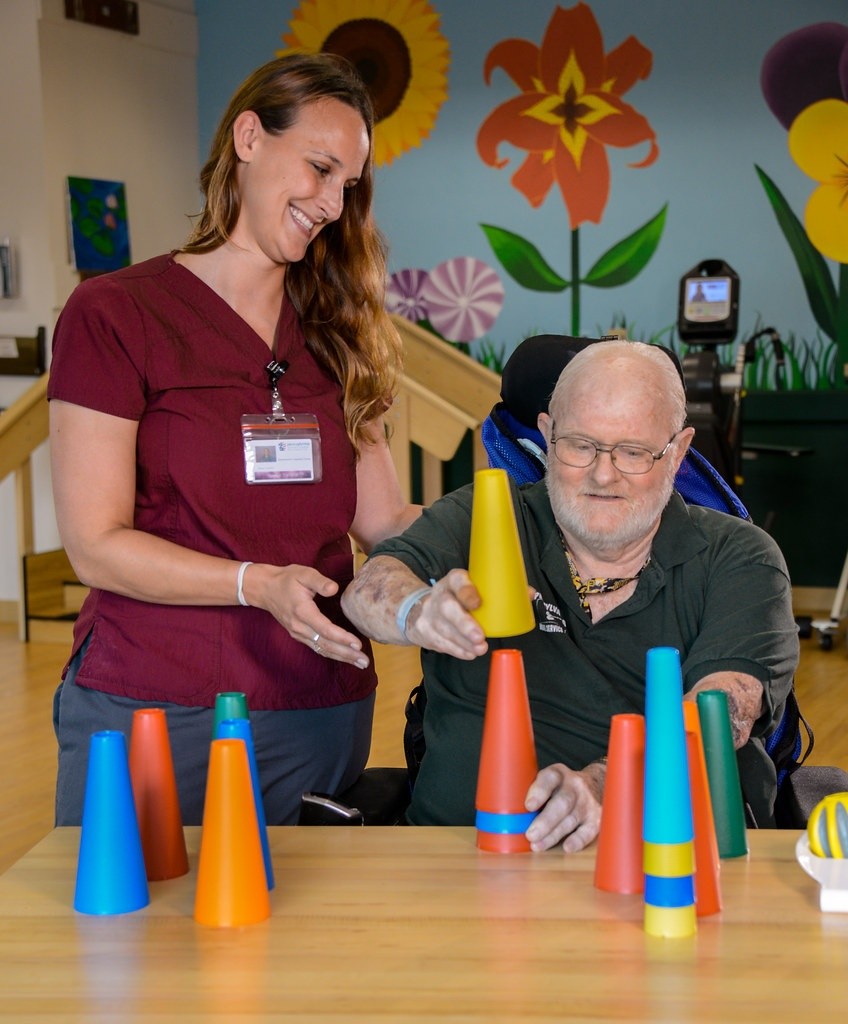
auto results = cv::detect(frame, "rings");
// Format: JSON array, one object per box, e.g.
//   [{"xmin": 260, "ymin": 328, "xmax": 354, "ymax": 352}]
[{"xmin": 313, "ymin": 633, "xmax": 320, "ymax": 642}]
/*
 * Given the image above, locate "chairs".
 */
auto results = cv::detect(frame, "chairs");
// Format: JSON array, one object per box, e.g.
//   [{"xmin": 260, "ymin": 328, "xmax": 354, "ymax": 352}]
[{"xmin": 300, "ymin": 334, "xmax": 848, "ymax": 831}]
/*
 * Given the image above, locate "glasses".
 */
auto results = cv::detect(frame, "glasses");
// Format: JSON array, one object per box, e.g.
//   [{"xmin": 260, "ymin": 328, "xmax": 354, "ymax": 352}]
[{"xmin": 550, "ymin": 419, "xmax": 676, "ymax": 475}]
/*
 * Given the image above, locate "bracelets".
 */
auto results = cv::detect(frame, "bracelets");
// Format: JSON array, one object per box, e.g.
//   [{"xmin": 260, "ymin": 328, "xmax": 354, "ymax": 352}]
[
  {"xmin": 596, "ymin": 755, "xmax": 607, "ymax": 765},
  {"xmin": 237, "ymin": 562, "xmax": 253, "ymax": 607},
  {"xmin": 397, "ymin": 586, "xmax": 432, "ymax": 633}
]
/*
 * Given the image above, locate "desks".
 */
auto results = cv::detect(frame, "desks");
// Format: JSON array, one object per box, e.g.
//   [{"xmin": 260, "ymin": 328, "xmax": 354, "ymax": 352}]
[{"xmin": 0, "ymin": 825, "xmax": 848, "ymax": 1024}]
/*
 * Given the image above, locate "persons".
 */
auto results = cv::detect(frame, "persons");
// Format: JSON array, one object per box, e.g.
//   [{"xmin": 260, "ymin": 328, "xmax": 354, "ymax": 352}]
[
  {"xmin": 262, "ymin": 448, "xmax": 275, "ymax": 461},
  {"xmin": 48, "ymin": 52, "xmax": 434, "ymax": 826},
  {"xmin": 339, "ymin": 340, "xmax": 801, "ymax": 855}
]
